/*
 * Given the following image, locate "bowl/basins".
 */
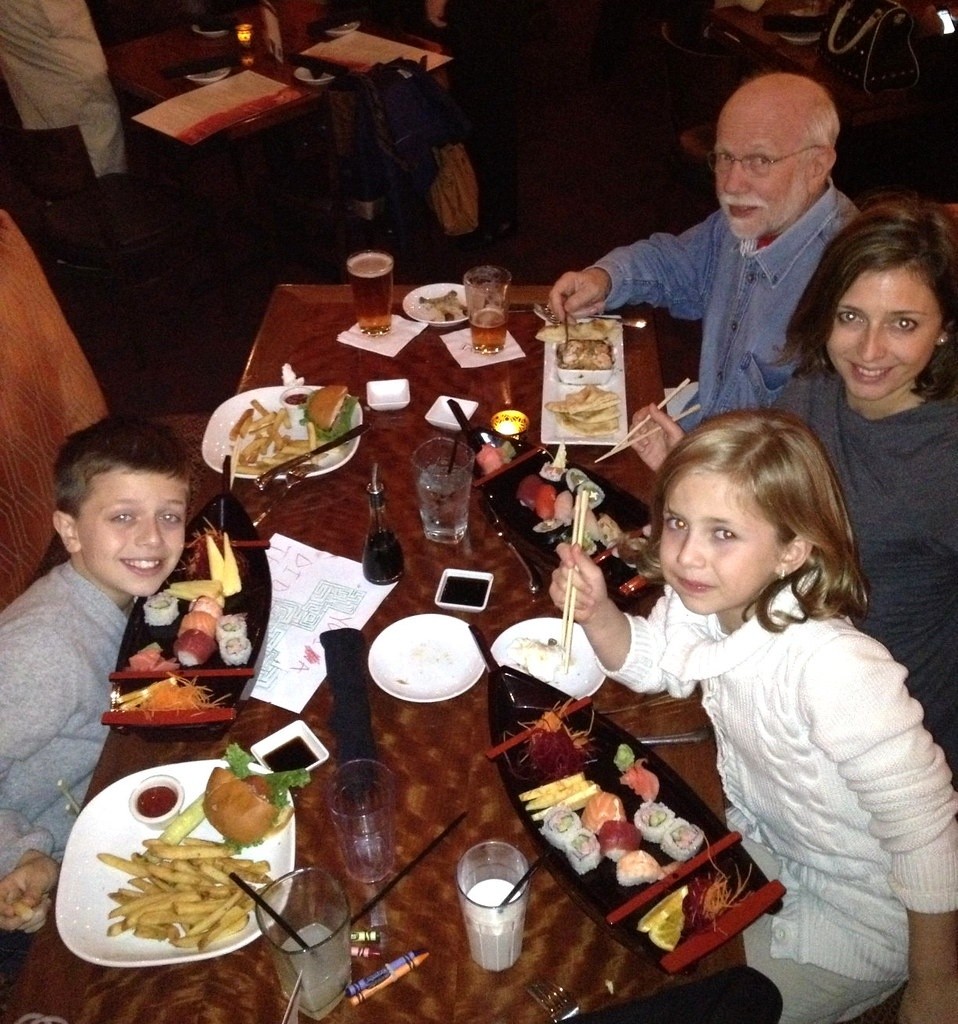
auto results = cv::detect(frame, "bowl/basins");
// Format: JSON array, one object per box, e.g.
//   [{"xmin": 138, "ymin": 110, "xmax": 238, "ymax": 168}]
[
  {"xmin": 555, "ymin": 339, "xmax": 614, "ymax": 385},
  {"xmin": 365, "ymin": 378, "xmax": 478, "ymax": 430},
  {"xmin": 281, "ymin": 387, "xmax": 314, "ymax": 420},
  {"xmin": 128, "ymin": 774, "xmax": 184, "ymax": 831},
  {"xmin": 249, "ymin": 719, "xmax": 330, "ymax": 773},
  {"xmin": 434, "ymin": 568, "xmax": 493, "ymax": 612}
]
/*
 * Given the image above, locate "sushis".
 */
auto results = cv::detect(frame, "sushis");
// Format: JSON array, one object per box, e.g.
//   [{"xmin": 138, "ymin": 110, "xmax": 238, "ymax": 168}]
[
  {"xmin": 518, "ymin": 465, "xmax": 606, "ymax": 557},
  {"xmin": 538, "ymin": 792, "xmax": 704, "ymax": 887},
  {"xmin": 142, "ymin": 591, "xmax": 254, "ymax": 668}
]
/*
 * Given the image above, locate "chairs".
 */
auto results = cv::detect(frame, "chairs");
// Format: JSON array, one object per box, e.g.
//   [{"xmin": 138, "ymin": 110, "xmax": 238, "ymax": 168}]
[{"xmin": 0, "ymin": 124, "xmax": 240, "ymax": 351}]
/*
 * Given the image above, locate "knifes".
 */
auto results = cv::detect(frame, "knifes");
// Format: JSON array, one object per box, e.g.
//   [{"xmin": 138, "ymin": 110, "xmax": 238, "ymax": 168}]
[{"xmin": 252, "ymin": 421, "xmax": 373, "ymax": 490}]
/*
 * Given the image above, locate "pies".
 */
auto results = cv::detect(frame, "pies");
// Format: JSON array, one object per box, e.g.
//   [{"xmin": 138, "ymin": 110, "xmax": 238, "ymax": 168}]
[{"xmin": 544, "ymin": 383, "xmax": 623, "ymax": 435}]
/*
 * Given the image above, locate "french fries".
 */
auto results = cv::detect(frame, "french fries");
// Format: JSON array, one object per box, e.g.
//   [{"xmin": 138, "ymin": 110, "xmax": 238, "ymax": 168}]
[
  {"xmin": 98, "ymin": 837, "xmax": 278, "ymax": 953},
  {"xmin": 229, "ymin": 398, "xmax": 317, "ymax": 474}
]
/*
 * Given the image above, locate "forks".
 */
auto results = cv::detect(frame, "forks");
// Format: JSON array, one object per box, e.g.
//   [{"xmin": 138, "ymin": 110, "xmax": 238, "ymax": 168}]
[
  {"xmin": 523, "ymin": 976, "xmax": 579, "ymax": 1024},
  {"xmin": 250, "ymin": 463, "xmax": 322, "ymax": 526}
]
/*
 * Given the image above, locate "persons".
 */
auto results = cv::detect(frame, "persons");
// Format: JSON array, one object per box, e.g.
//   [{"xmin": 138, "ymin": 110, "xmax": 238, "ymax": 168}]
[
  {"xmin": 0, "ymin": 1, "xmax": 521, "ymax": 286},
  {"xmin": 0, "ymin": 415, "xmax": 200, "ymax": 986},
  {"xmin": 550, "ymin": 72, "xmax": 865, "ymax": 434},
  {"xmin": 549, "ymin": 410, "xmax": 958, "ymax": 1024},
  {"xmin": 630, "ymin": 199, "xmax": 958, "ymax": 791}
]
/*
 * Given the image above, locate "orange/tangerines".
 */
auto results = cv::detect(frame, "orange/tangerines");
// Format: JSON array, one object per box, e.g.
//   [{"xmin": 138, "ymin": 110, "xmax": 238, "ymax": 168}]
[{"xmin": 636, "ymin": 886, "xmax": 689, "ymax": 953}]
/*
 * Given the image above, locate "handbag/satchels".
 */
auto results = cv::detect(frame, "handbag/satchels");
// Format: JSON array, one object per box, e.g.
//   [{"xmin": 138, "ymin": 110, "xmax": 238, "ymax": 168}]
[{"xmin": 815, "ymin": 0, "xmax": 919, "ymax": 96}]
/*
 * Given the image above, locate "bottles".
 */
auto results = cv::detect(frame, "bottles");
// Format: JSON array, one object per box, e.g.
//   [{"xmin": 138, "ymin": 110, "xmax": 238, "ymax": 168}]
[{"xmin": 361, "ymin": 463, "xmax": 405, "ymax": 585}]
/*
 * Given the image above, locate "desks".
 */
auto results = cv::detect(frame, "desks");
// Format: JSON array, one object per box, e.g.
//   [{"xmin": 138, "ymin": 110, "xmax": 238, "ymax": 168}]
[
  {"xmin": 711, "ymin": 0, "xmax": 958, "ymax": 132},
  {"xmin": 0, "ymin": 282, "xmax": 746, "ymax": 1024},
  {"xmin": 103, "ymin": 1, "xmax": 457, "ymax": 281}
]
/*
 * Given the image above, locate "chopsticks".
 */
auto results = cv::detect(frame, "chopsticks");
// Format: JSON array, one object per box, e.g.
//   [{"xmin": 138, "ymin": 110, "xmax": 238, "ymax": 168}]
[
  {"xmin": 561, "ymin": 490, "xmax": 589, "ymax": 672},
  {"xmin": 593, "ymin": 377, "xmax": 700, "ymax": 463}
]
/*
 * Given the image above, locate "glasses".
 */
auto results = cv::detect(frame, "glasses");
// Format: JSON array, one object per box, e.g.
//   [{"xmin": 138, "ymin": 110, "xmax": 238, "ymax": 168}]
[{"xmin": 708, "ymin": 145, "xmax": 826, "ymax": 178}]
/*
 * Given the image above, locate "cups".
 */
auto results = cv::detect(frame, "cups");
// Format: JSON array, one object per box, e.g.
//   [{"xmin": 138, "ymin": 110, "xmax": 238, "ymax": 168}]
[
  {"xmin": 454, "ymin": 841, "xmax": 530, "ymax": 971},
  {"xmin": 253, "ymin": 866, "xmax": 352, "ymax": 1020},
  {"xmin": 324, "ymin": 758, "xmax": 397, "ymax": 883},
  {"xmin": 409, "ymin": 436, "xmax": 476, "ymax": 545},
  {"xmin": 346, "ymin": 249, "xmax": 394, "ymax": 336},
  {"xmin": 464, "ymin": 267, "xmax": 512, "ymax": 355}
]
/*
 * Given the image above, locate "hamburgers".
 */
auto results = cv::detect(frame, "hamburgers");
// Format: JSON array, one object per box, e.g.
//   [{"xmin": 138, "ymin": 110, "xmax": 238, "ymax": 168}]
[
  {"xmin": 298, "ymin": 384, "xmax": 359, "ymax": 443},
  {"xmin": 205, "ymin": 743, "xmax": 311, "ymax": 853}
]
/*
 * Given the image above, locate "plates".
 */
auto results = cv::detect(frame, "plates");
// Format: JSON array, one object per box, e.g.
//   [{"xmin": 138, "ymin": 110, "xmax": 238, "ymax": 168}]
[
  {"xmin": 112, "ymin": 493, "xmax": 273, "ymax": 743},
  {"xmin": 402, "ymin": 282, "xmax": 469, "ymax": 327},
  {"xmin": 469, "ymin": 424, "xmax": 651, "ymax": 594},
  {"xmin": 190, "ymin": 22, "xmax": 360, "ymax": 84},
  {"xmin": 54, "ymin": 758, "xmax": 296, "ymax": 968},
  {"xmin": 367, "ymin": 614, "xmax": 485, "ymax": 704},
  {"xmin": 490, "ymin": 617, "xmax": 606, "ymax": 700},
  {"xmin": 539, "ymin": 314, "xmax": 629, "ymax": 446},
  {"xmin": 485, "ymin": 664, "xmax": 783, "ymax": 975},
  {"xmin": 201, "ymin": 385, "xmax": 362, "ymax": 478}
]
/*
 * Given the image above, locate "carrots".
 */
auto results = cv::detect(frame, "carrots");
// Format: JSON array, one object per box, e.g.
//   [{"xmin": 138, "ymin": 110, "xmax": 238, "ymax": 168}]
[
  {"xmin": 139, "ymin": 670, "xmax": 232, "ymax": 720},
  {"xmin": 514, "ymin": 698, "xmax": 595, "ymax": 751},
  {"xmin": 699, "ymin": 842, "xmax": 754, "ymax": 932}
]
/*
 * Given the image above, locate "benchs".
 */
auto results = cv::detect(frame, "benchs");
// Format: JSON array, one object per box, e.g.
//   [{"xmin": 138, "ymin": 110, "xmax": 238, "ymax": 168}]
[{"xmin": 0, "ymin": 209, "xmax": 206, "ymax": 618}]
